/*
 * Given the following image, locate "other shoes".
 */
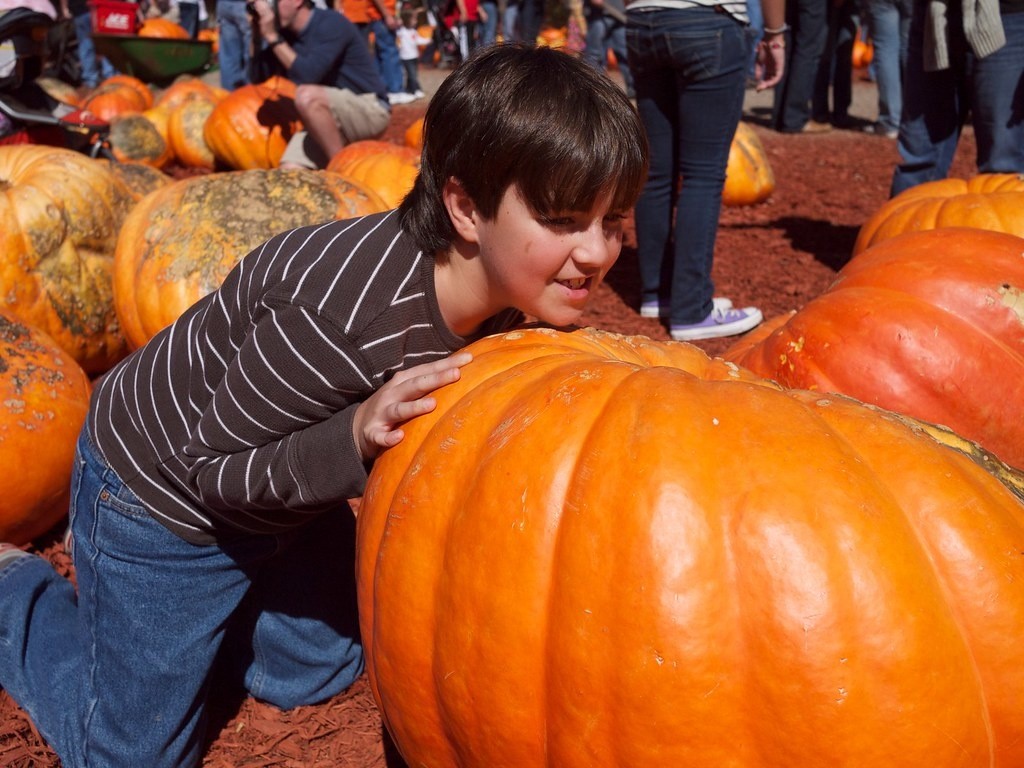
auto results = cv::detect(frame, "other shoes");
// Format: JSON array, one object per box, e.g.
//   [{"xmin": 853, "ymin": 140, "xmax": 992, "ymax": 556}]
[
  {"xmin": 798, "ymin": 118, "xmax": 830, "ymax": 133},
  {"xmin": 387, "ymin": 93, "xmax": 416, "ymax": 104},
  {"xmin": 864, "ymin": 123, "xmax": 898, "ymax": 138},
  {"xmin": 415, "ymin": 91, "xmax": 425, "ymax": 99}
]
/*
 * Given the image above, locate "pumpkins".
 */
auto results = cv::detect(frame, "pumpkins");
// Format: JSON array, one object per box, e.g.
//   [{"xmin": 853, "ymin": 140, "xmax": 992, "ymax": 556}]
[{"xmin": 0, "ymin": 15, "xmax": 1024, "ymax": 768}]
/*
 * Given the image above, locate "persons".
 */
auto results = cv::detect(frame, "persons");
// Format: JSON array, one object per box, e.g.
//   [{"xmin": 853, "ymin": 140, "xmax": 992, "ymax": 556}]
[
  {"xmin": 0, "ymin": 0, "xmax": 1023, "ymax": 343},
  {"xmin": 0, "ymin": 43, "xmax": 644, "ymax": 768}
]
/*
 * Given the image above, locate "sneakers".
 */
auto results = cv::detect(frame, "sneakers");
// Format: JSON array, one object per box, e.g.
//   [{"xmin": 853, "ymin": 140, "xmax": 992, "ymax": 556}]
[
  {"xmin": 670, "ymin": 306, "xmax": 761, "ymax": 341},
  {"xmin": 640, "ymin": 297, "xmax": 733, "ymax": 317}
]
